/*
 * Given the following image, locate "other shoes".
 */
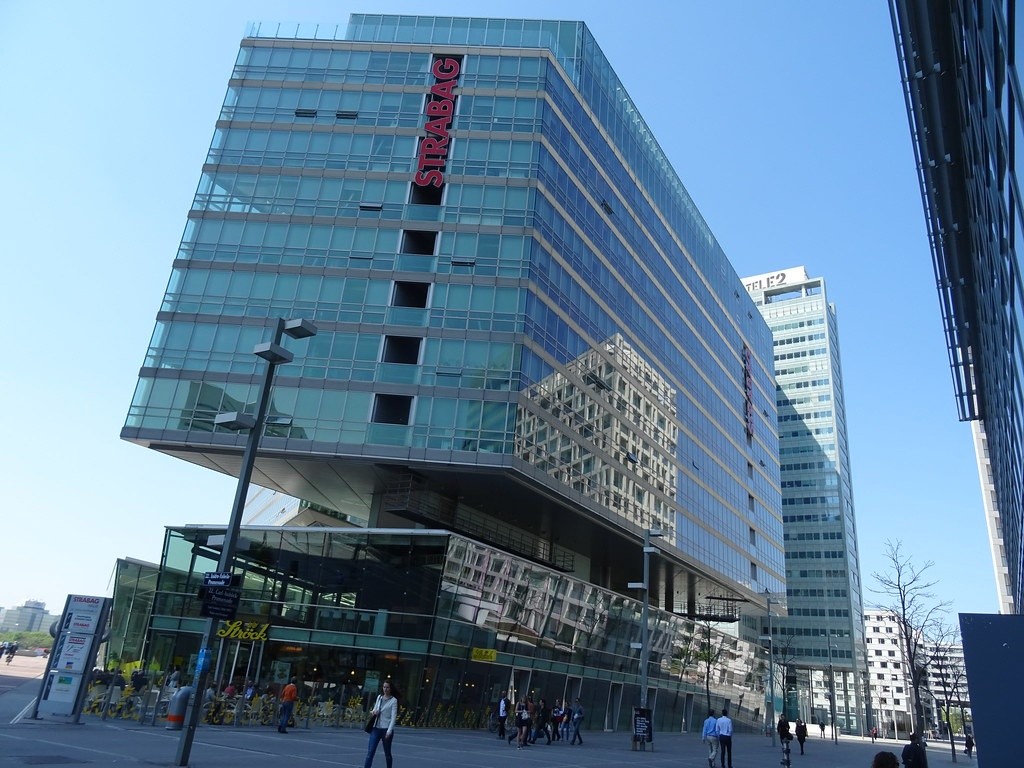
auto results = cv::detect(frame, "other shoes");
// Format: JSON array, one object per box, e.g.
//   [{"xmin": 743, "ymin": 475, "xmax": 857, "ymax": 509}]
[
  {"xmin": 517, "ymin": 745, "xmax": 523, "ymax": 750},
  {"xmin": 529, "ymin": 740, "xmax": 535, "ymax": 744},
  {"xmin": 281, "ymin": 730, "xmax": 288, "ymax": 734},
  {"xmin": 557, "ymin": 736, "xmax": 561, "ymax": 741},
  {"xmin": 524, "ymin": 743, "xmax": 531, "ymax": 746},
  {"xmin": 546, "ymin": 740, "xmax": 551, "ymax": 745},
  {"xmin": 708, "ymin": 758, "xmax": 712, "ymax": 768},
  {"xmin": 578, "ymin": 742, "xmax": 582, "ymax": 745},
  {"xmin": 508, "ymin": 736, "xmax": 511, "ymax": 744}
]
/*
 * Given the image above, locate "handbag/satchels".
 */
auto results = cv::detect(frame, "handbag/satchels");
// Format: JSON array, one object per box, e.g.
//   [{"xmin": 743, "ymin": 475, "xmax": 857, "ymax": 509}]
[
  {"xmin": 786, "ymin": 731, "xmax": 793, "ymax": 741},
  {"xmin": 365, "ymin": 714, "xmax": 376, "ymax": 733},
  {"xmin": 563, "ymin": 714, "xmax": 567, "ymax": 723},
  {"xmin": 521, "ymin": 710, "xmax": 528, "ymax": 720}
]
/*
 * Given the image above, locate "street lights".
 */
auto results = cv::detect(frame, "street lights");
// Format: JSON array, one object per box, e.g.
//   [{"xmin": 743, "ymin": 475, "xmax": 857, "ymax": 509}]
[
  {"xmin": 759, "ymin": 597, "xmax": 780, "ymax": 748},
  {"xmin": 626, "ymin": 529, "xmax": 662, "ymax": 752},
  {"xmin": 173, "ymin": 317, "xmax": 319, "ymax": 765},
  {"xmin": 863, "ymin": 679, "xmax": 874, "ymax": 744},
  {"xmin": 821, "ymin": 633, "xmax": 838, "ymax": 744}
]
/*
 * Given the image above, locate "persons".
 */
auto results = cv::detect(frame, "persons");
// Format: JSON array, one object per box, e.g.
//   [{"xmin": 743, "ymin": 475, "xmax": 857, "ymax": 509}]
[
  {"xmin": 0, "ymin": 640, "xmax": 6, "ymax": 659},
  {"xmin": 87, "ymin": 666, "xmax": 186, "ymax": 720},
  {"xmin": 278, "ymin": 676, "xmax": 299, "ymax": 733},
  {"xmin": 902, "ymin": 733, "xmax": 928, "ymax": 768},
  {"xmin": 364, "ymin": 680, "xmax": 397, "ymax": 768},
  {"xmin": 5, "ymin": 641, "xmax": 19, "ymax": 662},
  {"xmin": 777, "ymin": 714, "xmax": 790, "ymax": 746},
  {"xmin": 494, "ymin": 691, "xmax": 511, "ymax": 740},
  {"xmin": 202, "ymin": 680, "xmax": 277, "ymax": 725},
  {"xmin": 716, "ymin": 710, "xmax": 735, "ymax": 768},
  {"xmin": 872, "ymin": 751, "xmax": 899, "ymax": 768},
  {"xmin": 508, "ymin": 695, "xmax": 585, "ymax": 750},
  {"xmin": 795, "ymin": 719, "xmax": 805, "ymax": 755},
  {"xmin": 873, "ymin": 727, "xmax": 877, "ymax": 739},
  {"xmin": 820, "ymin": 722, "xmax": 825, "ymax": 739},
  {"xmin": 702, "ymin": 709, "xmax": 719, "ymax": 768},
  {"xmin": 703, "ymin": 713, "xmax": 708, "ymax": 726},
  {"xmin": 965, "ymin": 735, "xmax": 974, "ymax": 756}
]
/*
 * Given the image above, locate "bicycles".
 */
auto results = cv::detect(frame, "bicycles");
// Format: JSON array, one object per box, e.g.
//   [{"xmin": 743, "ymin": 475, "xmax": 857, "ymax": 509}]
[{"xmin": 488, "ymin": 708, "xmax": 514, "ymax": 733}]
